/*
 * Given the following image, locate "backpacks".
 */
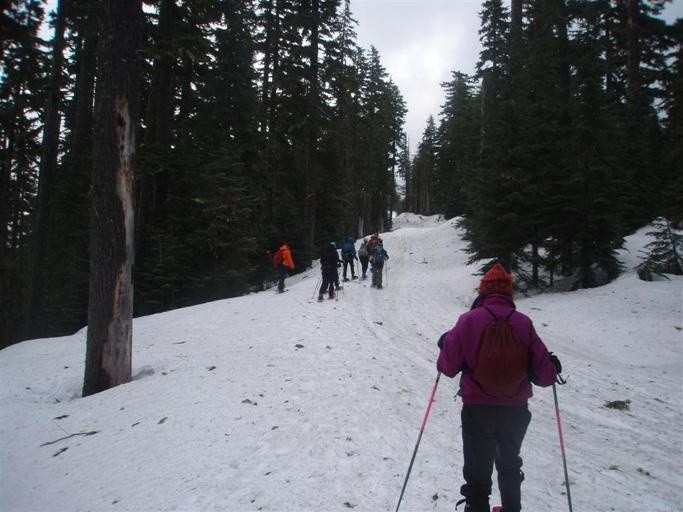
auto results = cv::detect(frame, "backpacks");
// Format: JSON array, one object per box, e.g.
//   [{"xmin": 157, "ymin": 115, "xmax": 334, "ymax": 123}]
[
  {"xmin": 358, "ymin": 243, "xmax": 368, "ymax": 258},
  {"xmin": 469, "ymin": 303, "xmax": 532, "ymax": 399},
  {"xmin": 271, "ymin": 250, "xmax": 284, "ymax": 266},
  {"xmin": 374, "ymin": 247, "xmax": 385, "ymax": 264},
  {"xmin": 342, "ymin": 243, "xmax": 354, "ymax": 253}
]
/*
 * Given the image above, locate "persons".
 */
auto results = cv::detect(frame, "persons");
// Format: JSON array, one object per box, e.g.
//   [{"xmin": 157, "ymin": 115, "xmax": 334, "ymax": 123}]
[
  {"xmin": 358, "ymin": 238, "xmax": 369, "ymax": 277},
  {"xmin": 318, "ymin": 245, "xmax": 337, "ymax": 300},
  {"xmin": 324, "ymin": 241, "xmax": 344, "ymax": 294},
  {"xmin": 340, "ymin": 237, "xmax": 357, "ymax": 282},
  {"xmin": 275, "ymin": 237, "xmax": 294, "ymax": 292},
  {"xmin": 366, "ymin": 232, "xmax": 378, "ymax": 268},
  {"xmin": 436, "ymin": 262, "xmax": 560, "ymax": 512},
  {"xmin": 369, "ymin": 242, "xmax": 388, "ymax": 288}
]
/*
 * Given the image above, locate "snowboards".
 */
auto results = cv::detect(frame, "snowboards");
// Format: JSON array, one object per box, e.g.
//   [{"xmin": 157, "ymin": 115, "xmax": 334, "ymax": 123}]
[{"xmin": 359, "ymin": 275, "xmax": 368, "ymax": 280}]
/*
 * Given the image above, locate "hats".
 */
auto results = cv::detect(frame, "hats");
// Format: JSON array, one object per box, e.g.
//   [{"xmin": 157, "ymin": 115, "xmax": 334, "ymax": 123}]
[
  {"xmin": 478, "ymin": 262, "xmax": 514, "ymax": 297},
  {"xmin": 347, "ymin": 237, "xmax": 355, "ymax": 243},
  {"xmin": 364, "ymin": 237, "xmax": 369, "ymax": 243}
]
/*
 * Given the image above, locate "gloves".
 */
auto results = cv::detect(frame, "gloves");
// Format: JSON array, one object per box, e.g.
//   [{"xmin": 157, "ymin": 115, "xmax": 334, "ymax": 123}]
[
  {"xmin": 548, "ymin": 350, "xmax": 562, "ymax": 374},
  {"xmin": 437, "ymin": 331, "xmax": 451, "ymax": 350}
]
